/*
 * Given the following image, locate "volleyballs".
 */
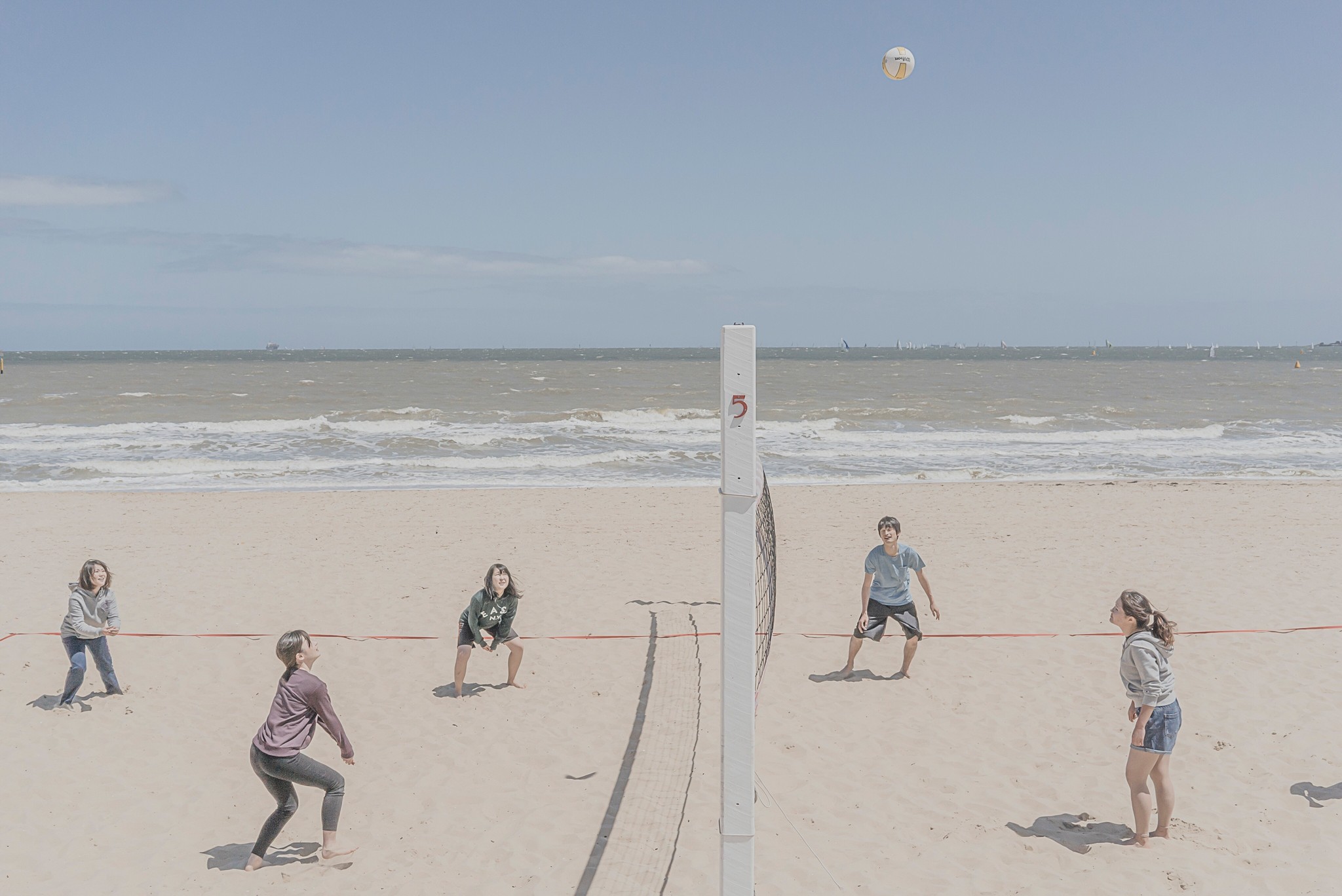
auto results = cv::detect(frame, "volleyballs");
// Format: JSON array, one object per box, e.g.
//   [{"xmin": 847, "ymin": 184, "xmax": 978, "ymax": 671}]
[{"xmin": 883, "ymin": 46, "xmax": 916, "ymax": 80}]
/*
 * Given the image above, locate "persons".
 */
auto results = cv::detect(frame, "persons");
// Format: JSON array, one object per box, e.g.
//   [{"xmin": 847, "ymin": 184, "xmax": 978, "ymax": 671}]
[
  {"xmin": 454, "ymin": 564, "xmax": 525, "ymax": 700},
  {"xmin": 60, "ymin": 559, "xmax": 123, "ymax": 705},
  {"xmin": 242, "ymin": 630, "xmax": 359, "ymax": 872},
  {"xmin": 836, "ymin": 517, "xmax": 941, "ymax": 678},
  {"xmin": 1109, "ymin": 591, "xmax": 1182, "ymax": 846}
]
